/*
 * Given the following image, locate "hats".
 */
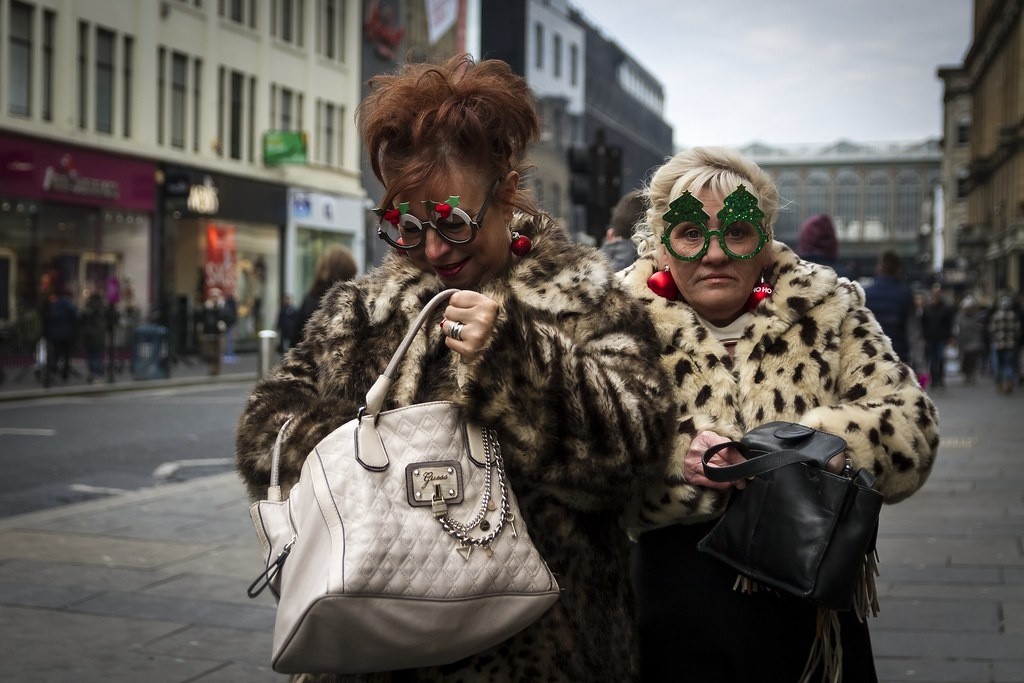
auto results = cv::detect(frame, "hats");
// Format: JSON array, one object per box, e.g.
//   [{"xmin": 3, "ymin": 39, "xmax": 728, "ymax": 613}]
[{"xmin": 797, "ymin": 215, "xmax": 839, "ymax": 262}]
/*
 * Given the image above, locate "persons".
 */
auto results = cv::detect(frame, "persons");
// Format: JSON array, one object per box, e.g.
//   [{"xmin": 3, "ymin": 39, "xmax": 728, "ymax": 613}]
[
  {"xmin": 864, "ymin": 249, "xmax": 916, "ymax": 365},
  {"xmin": 989, "ymin": 296, "xmax": 1020, "ymax": 392},
  {"xmin": 293, "ymin": 242, "xmax": 356, "ymax": 349},
  {"xmin": 955, "ymin": 296, "xmax": 985, "ymax": 384},
  {"xmin": 920, "ymin": 292, "xmax": 952, "ymax": 390},
  {"xmin": 601, "ymin": 188, "xmax": 651, "ymax": 273},
  {"xmin": 613, "ymin": 145, "xmax": 940, "ymax": 683},
  {"xmin": 38, "ymin": 279, "xmax": 170, "ymax": 384},
  {"xmin": 797, "ymin": 214, "xmax": 856, "ymax": 282},
  {"xmin": 278, "ymin": 293, "xmax": 297, "ymax": 356},
  {"xmin": 198, "ymin": 285, "xmax": 237, "ymax": 376},
  {"xmin": 238, "ymin": 55, "xmax": 675, "ymax": 683}
]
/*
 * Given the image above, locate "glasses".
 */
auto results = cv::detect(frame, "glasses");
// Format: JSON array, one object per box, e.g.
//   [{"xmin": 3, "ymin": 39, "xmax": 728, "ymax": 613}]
[
  {"xmin": 371, "ymin": 164, "xmax": 513, "ymax": 250},
  {"xmin": 660, "ymin": 183, "xmax": 772, "ymax": 262}
]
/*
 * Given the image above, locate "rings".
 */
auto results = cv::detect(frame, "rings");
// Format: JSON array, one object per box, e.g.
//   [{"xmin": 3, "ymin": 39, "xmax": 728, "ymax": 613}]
[{"xmin": 448, "ymin": 322, "xmax": 463, "ymax": 339}]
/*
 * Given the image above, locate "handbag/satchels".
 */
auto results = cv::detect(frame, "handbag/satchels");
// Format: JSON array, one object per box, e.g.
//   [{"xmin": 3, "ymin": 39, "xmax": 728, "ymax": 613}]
[
  {"xmin": 243, "ymin": 288, "xmax": 560, "ymax": 673},
  {"xmin": 697, "ymin": 421, "xmax": 886, "ymax": 604}
]
